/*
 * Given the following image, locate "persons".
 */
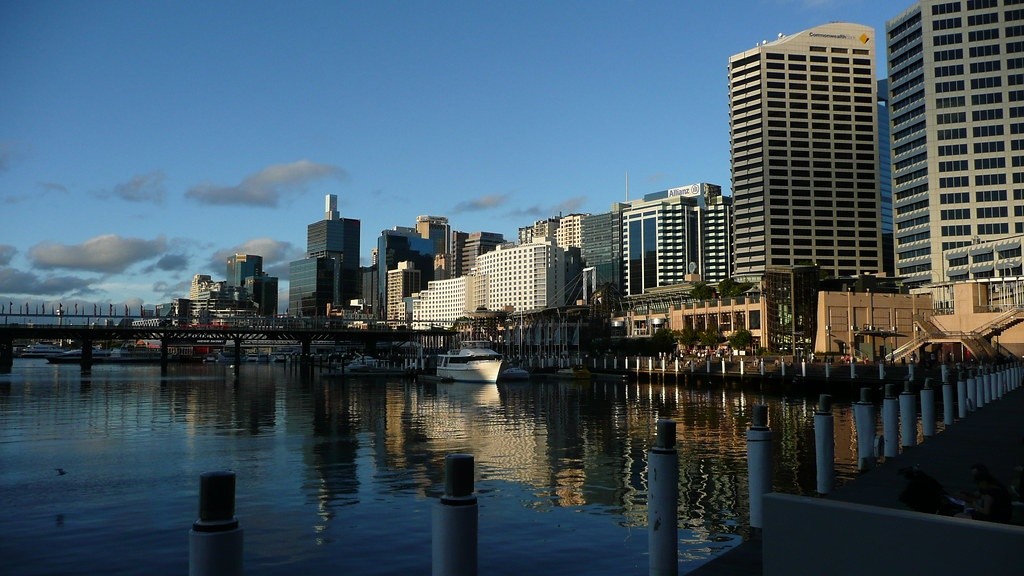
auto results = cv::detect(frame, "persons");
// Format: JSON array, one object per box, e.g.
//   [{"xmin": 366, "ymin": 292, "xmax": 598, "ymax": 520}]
[
  {"xmin": 680, "ymin": 345, "xmax": 868, "ymax": 372},
  {"xmin": 891, "ymin": 352, "xmax": 894, "ymax": 364},
  {"xmin": 909, "ymin": 351, "xmax": 918, "ymax": 363}
]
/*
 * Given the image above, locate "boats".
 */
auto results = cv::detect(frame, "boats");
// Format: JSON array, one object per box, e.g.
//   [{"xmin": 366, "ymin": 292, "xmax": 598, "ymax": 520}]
[
  {"xmin": 507, "ymin": 368, "xmax": 530, "ymax": 381},
  {"xmin": 19, "ymin": 343, "xmax": 64, "ymax": 358},
  {"xmin": 347, "ymin": 356, "xmax": 377, "ymax": 372},
  {"xmin": 558, "ymin": 365, "xmax": 591, "ymax": 379},
  {"xmin": 45, "ymin": 348, "xmax": 114, "ymax": 365},
  {"xmin": 436, "ymin": 340, "xmax": 504, "ymax": 384}
]
